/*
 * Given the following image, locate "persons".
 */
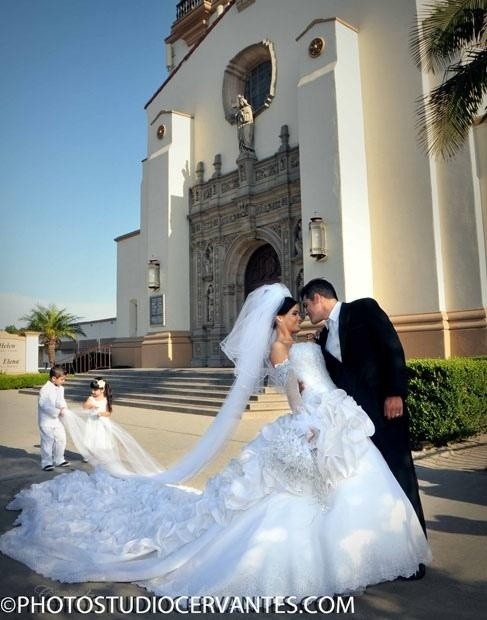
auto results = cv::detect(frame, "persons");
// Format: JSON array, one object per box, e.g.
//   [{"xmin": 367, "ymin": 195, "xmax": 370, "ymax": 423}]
[
  {"xmin": 224, "ymin": 283, "xmax": 430, "ymax": 600},
  {"xmin": 37, "ymin": 363, "xmax": 71, "ymax": 472},
  {"xmin": 297, "ymin": 278, "xmax": 428, "ymax": 585},
  {"xmin": 79, "ymin": 375, "xmax": 113, "ymax": 462},
  {"xmin": 232, "ymin": 94, "xmax": 256, "ymax": 162}
]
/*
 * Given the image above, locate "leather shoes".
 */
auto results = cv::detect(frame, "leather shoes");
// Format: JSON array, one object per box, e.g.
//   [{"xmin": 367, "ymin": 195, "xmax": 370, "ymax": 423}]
[{"xmin": 399, "ymin": 564, "xmax": 425, "ymax": 581}]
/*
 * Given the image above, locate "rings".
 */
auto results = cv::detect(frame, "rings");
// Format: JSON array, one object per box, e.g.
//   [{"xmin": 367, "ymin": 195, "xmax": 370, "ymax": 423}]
[{"xmin": 395, "ymin": 413, "xmax": 399, "ymax": 416}]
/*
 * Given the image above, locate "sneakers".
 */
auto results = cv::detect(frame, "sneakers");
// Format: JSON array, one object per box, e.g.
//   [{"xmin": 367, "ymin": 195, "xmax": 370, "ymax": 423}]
[
  {"xmin": 60, "ymin": 461, "xmax": 70, "ymax": 466},
  {"xmin": 43, "ymin": 465, "xmax": 55, "ymax": 471}
]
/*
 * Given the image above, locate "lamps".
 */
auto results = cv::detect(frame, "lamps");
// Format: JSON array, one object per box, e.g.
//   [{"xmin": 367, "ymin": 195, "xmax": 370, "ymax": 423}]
[
  {"xmin": 147, "ymin": 254, "xmax": 160, "ymax": 288},
  {"xmin": 309, "ymin": 209, "xmax": 327, "ymax": 257}
]
